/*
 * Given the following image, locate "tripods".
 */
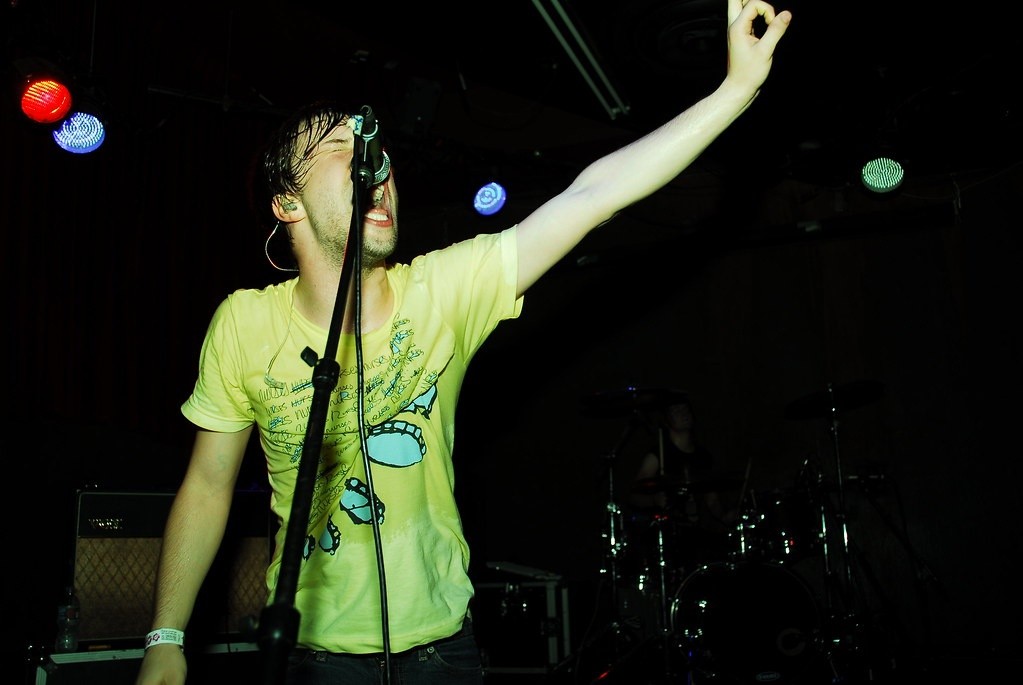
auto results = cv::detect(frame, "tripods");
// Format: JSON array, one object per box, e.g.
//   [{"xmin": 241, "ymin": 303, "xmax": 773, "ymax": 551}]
[{"xmin": 544, "ymin": 406, "xmax": 690, "ymax": 685}]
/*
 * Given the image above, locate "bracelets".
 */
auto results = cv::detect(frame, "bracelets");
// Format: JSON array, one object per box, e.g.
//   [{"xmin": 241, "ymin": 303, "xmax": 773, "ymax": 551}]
[{"xmin": 144, "ymin": 628, "xmax": 184, "ymax": 649}]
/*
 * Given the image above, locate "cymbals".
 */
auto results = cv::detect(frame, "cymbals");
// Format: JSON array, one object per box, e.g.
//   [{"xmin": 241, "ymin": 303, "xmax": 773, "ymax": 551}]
[
  {"xmin": 582, "ymin": 387, "xmax": 690, "ymax": 420},
  {"xmin": 782, "ymin": 379, "xmax": 885, "ymax": 422},
  {"xmin": 679, "ymin": 476, "xmax": 737, "ymax": 496}
]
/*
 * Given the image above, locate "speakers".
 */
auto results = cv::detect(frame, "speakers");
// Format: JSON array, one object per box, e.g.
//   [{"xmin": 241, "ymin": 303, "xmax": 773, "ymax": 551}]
[{"xmin": 68, "ymin": 488, "xmax": 280, "ymax": 649}]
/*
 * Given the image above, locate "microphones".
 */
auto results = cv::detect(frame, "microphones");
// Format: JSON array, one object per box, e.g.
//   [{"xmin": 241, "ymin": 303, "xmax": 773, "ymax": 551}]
[
  {"xmin": 844, "ymin": 474, "xmax": 886, "ymax": 483},
  {"xmin": 355, "ymin": 105, "xmax": 391, "ymax": 186}
]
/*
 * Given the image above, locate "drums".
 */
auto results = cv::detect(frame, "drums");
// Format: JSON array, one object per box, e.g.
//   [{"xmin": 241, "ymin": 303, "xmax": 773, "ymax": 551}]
[
  {"xmin": 741, "ymin": 473, "xmax": 840, "ymax": 563},
  {"xmin": 600, "ymin": 510, "xmax": 686, "ymax": 587},
  {"xmin": 669, "ymin": 556, "xmax": 822, "ymax": 685}
]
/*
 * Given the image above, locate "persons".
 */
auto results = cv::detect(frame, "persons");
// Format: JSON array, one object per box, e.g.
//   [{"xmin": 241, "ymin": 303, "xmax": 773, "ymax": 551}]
[
  {"xmin": 136, "ymin": 0, "xmax": 792, "ymax": 684},
  {"xmin": 633, "ymin": 395, "xmax": 741, "ymax": 524}
]
